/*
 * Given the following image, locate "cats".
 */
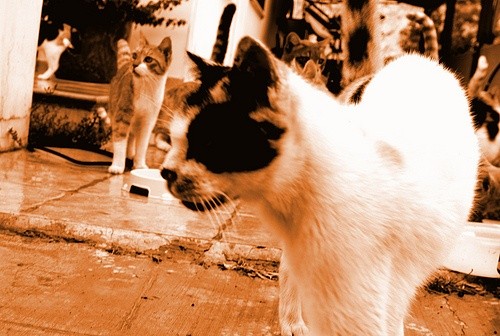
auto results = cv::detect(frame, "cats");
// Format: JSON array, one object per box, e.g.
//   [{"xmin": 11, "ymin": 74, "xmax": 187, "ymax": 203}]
[
  {"xmin": 35, "ymin": 16, "xmax": 73, "ymax": 80},
  {"xmin": 104, "ymin": 4, "xmax": 340, "ymax": 175},
  {"xmin": 158, "ymin": 36, "xmax": 482, "ymax": 336}
]
